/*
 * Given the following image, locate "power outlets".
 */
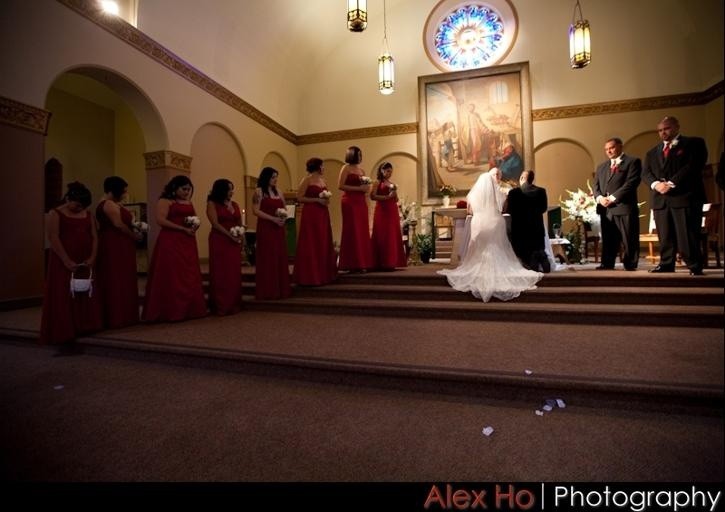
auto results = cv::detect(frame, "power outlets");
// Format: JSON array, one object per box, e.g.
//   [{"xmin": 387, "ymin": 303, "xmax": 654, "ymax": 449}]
[{"xmin": 569, "ymin": 1, "xmax": 592, "ymax": 70}]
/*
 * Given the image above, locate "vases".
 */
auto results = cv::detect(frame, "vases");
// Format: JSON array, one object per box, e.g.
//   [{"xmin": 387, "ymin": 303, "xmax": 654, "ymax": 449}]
[{"xmin": 443, "ymin": 195, "xmax": 449, "ymax": 206}]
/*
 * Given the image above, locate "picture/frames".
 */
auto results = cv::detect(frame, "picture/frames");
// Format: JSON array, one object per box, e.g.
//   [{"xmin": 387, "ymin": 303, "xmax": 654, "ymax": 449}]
[{"xmin": 416, "ymin": 60, "xmax": 536, "ymax": 207}]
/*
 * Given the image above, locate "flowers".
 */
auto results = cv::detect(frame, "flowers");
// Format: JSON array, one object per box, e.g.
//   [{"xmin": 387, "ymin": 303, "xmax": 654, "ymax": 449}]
[
  {"xmin": 317, "ymin": 189, "xmax": 331, "ymax": 201},
  {"xmin": 389, "ymin": 182, "xmax": 398, "ymax": 194},
  {"xmin": 361, "ymin": 174, "xmax": 371, "ymax": 186},
  {"xmin": 438, "ymin": 182, "xmax": 456, "ymax": 198},
  {"xmin": 184, "ymin": 214, "xmax": 201, "ymax": 233},
  {"xmin": 276, "ymin": 207, "xmax": 287, "ymax": 223},
  {"xmin": 548, "ymin": 176, "xmax": 647, "ymax": 265},
  {"xmin": 229, "ymin": 225, "xmax": 245, "ymax": 246}
]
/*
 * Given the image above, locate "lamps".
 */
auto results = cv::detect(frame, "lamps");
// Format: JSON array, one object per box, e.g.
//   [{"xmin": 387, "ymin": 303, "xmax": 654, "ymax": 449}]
[
  {"xmin": 376, "ymin": 1, "xmax": 397, "ymax": 96},
  {"xmin": 347, "ymin": 0, "xmax": 367, "ymax": 33}
]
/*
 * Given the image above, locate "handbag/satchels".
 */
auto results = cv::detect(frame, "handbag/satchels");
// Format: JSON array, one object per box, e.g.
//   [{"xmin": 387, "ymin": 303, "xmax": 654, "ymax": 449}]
[{"xmin": 71, "ymin": 277, "xmax": 92, "ymax": 297}]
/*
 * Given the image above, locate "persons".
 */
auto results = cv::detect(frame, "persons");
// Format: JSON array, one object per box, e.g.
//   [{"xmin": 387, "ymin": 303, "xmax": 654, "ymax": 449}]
[
  {"xmin": 369, "ymin": 162, "xmax": 408, "ymax": 271},
  {"xmin": 439, "ymin": 103, "xmax": 522, "ymax": 181},
  {"xmin": 40, "ymin": 183, "xmax": 101, "ymax": 356},
  {"xmin": 466, "ymin": 172, "xmax": 507, "ymax": 273},
  {"xmin": 593, "ymin": 136, "xmax": 642, "ymax": 270},
  {"xmin": 337, "ymin": 147, "xmax": 373, "ymax": 273},
  {"xmin": 640, "ymin": 117, "xmax": 707, "ymax": 275},
  {"xmin": 489, "ymin": 168, "xmax": 513, "ymax": 194},
  {"xmin": 206, "ymin": 178, "xmax": 245, "ymax": 316},
  {"xmin": 251, "ymin": 166, "xmax": 292, "ymax": 300},
  {"xmin": 95, "ymin": 176, "xmax": 145, "ymax": 330},
  {"xmin": 507, "ymin": 170, "xmax": 551, "ymax": 273},
  {"xmin": 291, "ymin": 157, "xmax": 337, "ymax": 286},
  {"xmin": 142, "ymin": 175, "xmax": 206, "ymax": 321}
]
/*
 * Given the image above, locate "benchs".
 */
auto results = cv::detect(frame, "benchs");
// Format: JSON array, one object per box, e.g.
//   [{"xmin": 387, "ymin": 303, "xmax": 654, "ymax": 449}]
[{"xmin": 638, "ymin": 202, "xmax": 723, "ymax": 265}]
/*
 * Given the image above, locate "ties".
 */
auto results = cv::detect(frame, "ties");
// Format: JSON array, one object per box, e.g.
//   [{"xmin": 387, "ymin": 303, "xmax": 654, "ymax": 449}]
[
  {"xmin": 611, "ymin": 161, "xmax": 615, "ymax": 172},
  {"xmin": 663, "ymin": 142, "xmax": 670, "ymax": 157}
]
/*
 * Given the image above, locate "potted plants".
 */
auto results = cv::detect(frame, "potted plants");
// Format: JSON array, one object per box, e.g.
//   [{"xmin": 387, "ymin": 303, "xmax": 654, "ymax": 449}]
[{"xmin": 413, "ymin": 229, "xmax": 435, "ymax": 264}]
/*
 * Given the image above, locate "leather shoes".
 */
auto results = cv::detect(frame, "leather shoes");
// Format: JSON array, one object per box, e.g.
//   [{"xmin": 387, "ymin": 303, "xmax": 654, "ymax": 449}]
[
  {"xmin": 596, "ymin": 265, "xmax": 614, "ymax": 270},
  {"xmin": 648, "ymin": 265, "xmax": 676, "ymax": 272}
]
